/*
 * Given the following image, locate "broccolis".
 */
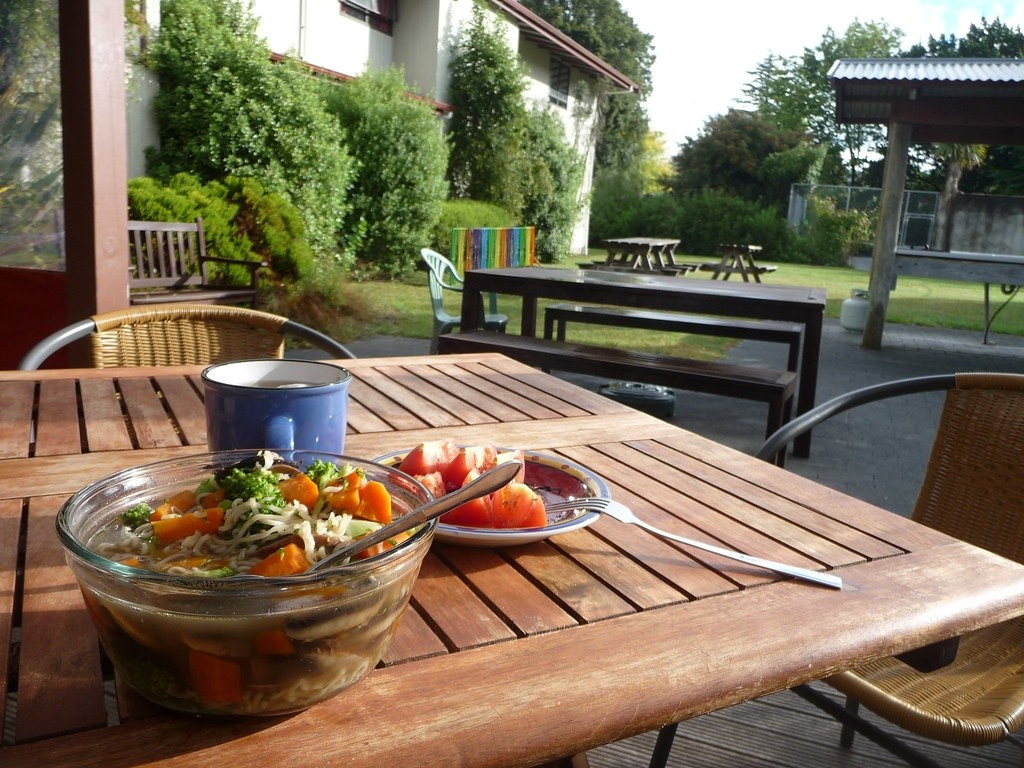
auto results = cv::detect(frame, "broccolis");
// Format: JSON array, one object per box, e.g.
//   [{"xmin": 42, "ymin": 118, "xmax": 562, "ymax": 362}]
[{"xmin": 124, "ymin": 457, "xmax": 339, "ymax": 576}]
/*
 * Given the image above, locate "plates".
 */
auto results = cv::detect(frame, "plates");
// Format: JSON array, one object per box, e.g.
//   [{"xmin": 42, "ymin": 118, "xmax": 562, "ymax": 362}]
[{"xmin": 366, "ymin": 446, "xmax": 610, "ymax": 546}]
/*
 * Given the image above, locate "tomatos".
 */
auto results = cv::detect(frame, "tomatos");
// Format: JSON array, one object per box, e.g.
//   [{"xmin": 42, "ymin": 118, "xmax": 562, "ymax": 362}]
[{"xmin": 389, "ymin": 436, "xmax": 549, "ymax": 528}]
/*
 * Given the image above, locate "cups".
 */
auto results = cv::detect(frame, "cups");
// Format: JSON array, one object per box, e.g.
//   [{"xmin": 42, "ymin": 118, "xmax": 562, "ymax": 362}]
[{"xmin": 201, "ymin": 358, "xmax": 352, "ymax": 485}]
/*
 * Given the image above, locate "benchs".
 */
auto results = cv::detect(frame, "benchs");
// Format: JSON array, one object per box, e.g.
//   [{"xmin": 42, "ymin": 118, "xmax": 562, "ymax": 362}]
[
  {"xmin": 52, "ymin": 207, "xmax": 268, "ymax": 314},
  {"xmin": 433, "ymin": 331, "xmax": 797, "ymax": 472},
  {"xmin": 575, "ymin": 260, "xmax": 778, "ymax": 276}
]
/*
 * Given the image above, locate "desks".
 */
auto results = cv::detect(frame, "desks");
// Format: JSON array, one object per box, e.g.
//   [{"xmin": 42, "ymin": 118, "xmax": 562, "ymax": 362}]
[
  {"xmin": 460, "ymin": 266, "xmax": 828, "ymax": 459},
  {"xmin": 0, "ymin": 353, "xmax": 1024, "ymax": 768},
  {"xmin": 601, "ymin": 237, "xmax": 681, "ymax": 269},
  {"xmin": 711, "ymin": 243, "xmax": 763, "ymax": 284},
  {"xmin": 543, "ymin": 303, "xmax": 801, "ymax": 421}
]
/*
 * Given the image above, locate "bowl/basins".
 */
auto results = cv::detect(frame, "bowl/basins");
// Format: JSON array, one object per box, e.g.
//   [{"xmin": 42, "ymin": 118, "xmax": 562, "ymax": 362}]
[{"xmin": 53, "ymin": 449, "xmax": 438, "ymax": 715}]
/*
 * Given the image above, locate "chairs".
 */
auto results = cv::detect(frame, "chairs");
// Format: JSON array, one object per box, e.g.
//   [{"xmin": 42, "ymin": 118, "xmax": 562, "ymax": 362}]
[
  {"xmin": 419, "ymin": 246, "xmax": 508, "ymax": 359},
  {"xmin": 643, "ymin": 372, "xmax": 1024, "ymax": 768},
  {"xmin": 18, "ymin": 303, "xmax": 358, "ymax": 370}
]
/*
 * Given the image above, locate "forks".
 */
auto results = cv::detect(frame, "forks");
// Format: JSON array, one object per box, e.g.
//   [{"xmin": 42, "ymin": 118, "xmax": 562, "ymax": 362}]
[{"xmin": 544, "ymin": 498, "xmax": 842, "ymax": 591}]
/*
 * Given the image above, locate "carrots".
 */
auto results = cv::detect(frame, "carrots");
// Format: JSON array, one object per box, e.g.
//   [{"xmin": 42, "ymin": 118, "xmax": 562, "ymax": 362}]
[{"xmin": 120, "ymin": 468, "xmax": 394, "ymax": 576}]
